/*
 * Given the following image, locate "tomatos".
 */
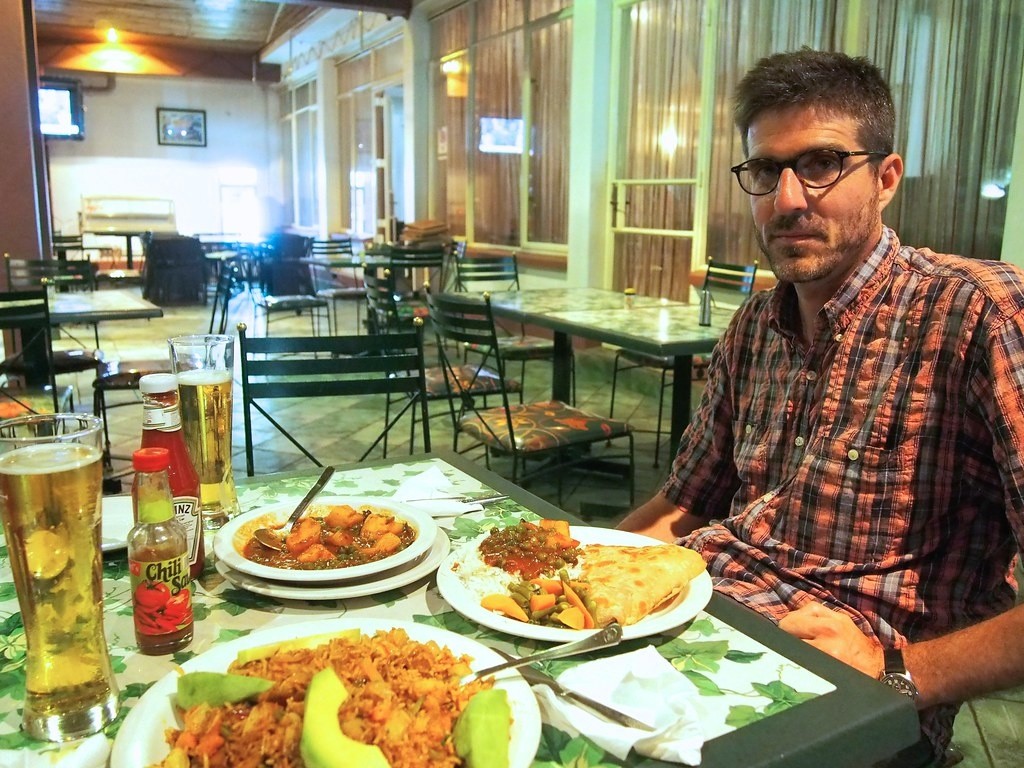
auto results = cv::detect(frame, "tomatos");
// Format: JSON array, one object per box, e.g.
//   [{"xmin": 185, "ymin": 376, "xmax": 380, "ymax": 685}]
[
  {"xmin": 135, "ymin": 580, "xmax": 170, "ymax": 607},
  {"xmin": 165, "ymin": 589, "xmax": 189, "ymax": 610}
]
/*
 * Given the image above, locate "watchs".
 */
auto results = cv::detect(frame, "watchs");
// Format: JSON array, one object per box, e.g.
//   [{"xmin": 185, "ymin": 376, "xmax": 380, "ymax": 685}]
[{"xmin": 879, "ymin": 649, "xmax": 919, "ymax": 701}]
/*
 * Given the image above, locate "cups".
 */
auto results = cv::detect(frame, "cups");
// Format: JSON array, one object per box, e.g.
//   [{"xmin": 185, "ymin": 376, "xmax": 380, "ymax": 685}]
[
  {"xmin": 0, "ymin": 413, "xmax": 122, "ymax": 744},
  {"xmin": 165, "ymin": 334, "xmax": 242, "ymax": 531}
]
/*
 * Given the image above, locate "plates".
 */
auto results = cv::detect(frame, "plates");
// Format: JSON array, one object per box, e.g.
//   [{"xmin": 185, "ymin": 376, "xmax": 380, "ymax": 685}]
[
  {"xmin": 437, "ymin": 525, "xmax": 713, "ymax": 642},
  {"xmin": 212, "ymin": 496, "xmax": 437, "ymax": 582},
  {"xmin": 100, "ymin": 495, "xmax": 133, "ymax": 552},
  {"xmin": 110, "ymin": 618, "xmax": 543, "ymax": 767},
  {"xmin": 215, "ymin": 526, "xmax": 451, "ymax": 600}
]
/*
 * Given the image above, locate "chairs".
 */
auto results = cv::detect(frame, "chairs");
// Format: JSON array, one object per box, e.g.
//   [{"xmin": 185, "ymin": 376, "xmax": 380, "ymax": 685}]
[
  {"xmin": 604, "ymin": 255, "xmax": 760, "ymax": 471},
  {"xmin": 453, "ymin": 250, "xmax": 577, "ymax": 410},
  {"xmin": 91, "ymin": 255, "xmax": 237, "ymax": 474},
  {"xmin": 0, "ymin": 276, "xmax": 75, "ymax": 451},
  {"xmin": 235, "ymin": 315, "xmax": 433, "ymax": 479},
  {"xmin": 422, "ymin": 280, "xmax": 636, "ymax": 515},
  {"xmin": 3, "ymin": 252, "xmax": 102, "ymax": 418},
  {"xmin": 47, "ymin": 214, "xmax": 474, "ymax": 335},
  {"xmin": 359, "ymin": 262, "xmax": 521, "ymax": 461}
]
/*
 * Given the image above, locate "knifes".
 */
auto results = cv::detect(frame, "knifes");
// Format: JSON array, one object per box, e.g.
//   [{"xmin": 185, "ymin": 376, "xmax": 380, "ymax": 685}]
[{"xmin": 488, "ymin": 646, "xmax": 658, "ymax": 733}]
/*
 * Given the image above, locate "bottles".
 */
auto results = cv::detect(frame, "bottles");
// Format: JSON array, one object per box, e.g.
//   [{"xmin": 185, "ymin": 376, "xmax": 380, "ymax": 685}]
[
  {"xmin": 128, "ymin": 449, "xmax": 194, "ymax": 656},
  {"xmin": 699, "ymin": 289, "xmax": 711, "ymax": 326},
  {"xmin": 623, "ymin": 288, "xmax": 636, "ymax": 310},
  {"xmin": 139, "ymin": 373, "xmax": 205, "ymax": 580}
]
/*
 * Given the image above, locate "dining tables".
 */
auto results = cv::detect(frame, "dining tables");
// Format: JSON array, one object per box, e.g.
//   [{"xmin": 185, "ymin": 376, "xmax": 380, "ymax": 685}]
[
  {"xmin": 446, "ymin": 285, "xmax": 693, "ymax": 492},
  {"xmin": 0, "ymin": 446, "xmax": 922, "ymax": 768},
  {"xmin": 0, "ymin": 289, "xmax": 164, "ymax": 472},
  {"xmin": 297, "ymin": 254, "xmax": 444, "ymax": 357},
  {"xmin": 527, "ymin": 303, "xmax": 742, "ymax": 480}
]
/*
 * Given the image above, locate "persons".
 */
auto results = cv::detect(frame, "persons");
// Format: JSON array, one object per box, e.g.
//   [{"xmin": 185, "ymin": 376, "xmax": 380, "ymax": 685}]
[{"xmin": 611, "ymin": 44, "xmax": 1023, "ymax": 768}]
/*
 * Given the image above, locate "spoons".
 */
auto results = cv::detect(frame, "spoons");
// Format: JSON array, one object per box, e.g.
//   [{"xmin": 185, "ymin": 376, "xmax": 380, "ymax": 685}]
[{"xmin": 253, "ymin": 465, "xmax": 335, "ymax": 551}]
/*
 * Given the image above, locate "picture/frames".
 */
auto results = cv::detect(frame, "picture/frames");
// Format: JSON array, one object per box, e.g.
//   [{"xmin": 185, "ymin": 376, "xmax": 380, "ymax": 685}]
[{"xmin": 156, "ymin": 108, "xmax": 207, "ymax": 147}]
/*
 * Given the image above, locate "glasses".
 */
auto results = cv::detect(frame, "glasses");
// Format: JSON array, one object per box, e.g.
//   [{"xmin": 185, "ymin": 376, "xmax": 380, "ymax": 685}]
[{"xmin": 731, "ymin": 148, "xmax": 889, "ymax": 196}]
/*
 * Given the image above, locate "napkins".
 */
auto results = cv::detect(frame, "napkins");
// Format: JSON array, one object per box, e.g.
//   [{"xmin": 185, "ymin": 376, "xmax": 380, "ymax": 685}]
[{"xmin": 530, "ymin": 645, "xmax": 736, "ymax": 766}]
